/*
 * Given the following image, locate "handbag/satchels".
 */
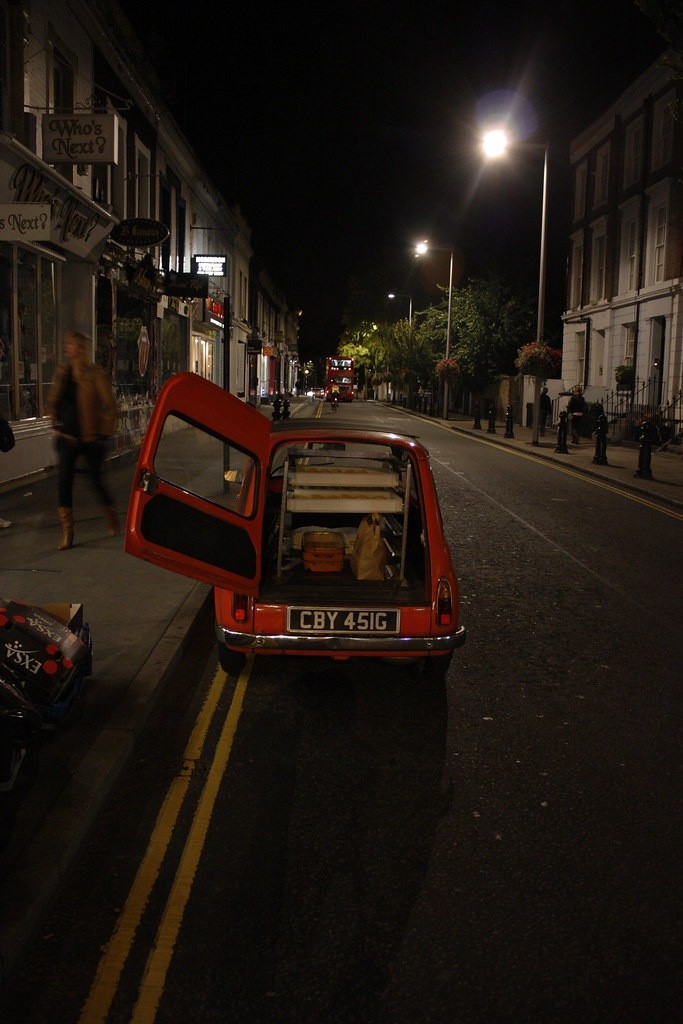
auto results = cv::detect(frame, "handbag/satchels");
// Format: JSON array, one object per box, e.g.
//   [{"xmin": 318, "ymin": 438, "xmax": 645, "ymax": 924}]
[
  {"xmin": 348, "ymin": 513, "xmax": 386, "ymax": 582},
  {"xmin": 0, "ymin": 419, "xmax": 16, "ymax": 453}
]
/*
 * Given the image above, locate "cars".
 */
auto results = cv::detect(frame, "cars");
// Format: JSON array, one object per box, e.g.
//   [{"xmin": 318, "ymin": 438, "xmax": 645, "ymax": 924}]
[
  {"xmin": 122, "ymin": 372, "xmax": 468, "ymax": 682},
  {"xmin": 353, "ymin": 389, "xmax": 358, "ymax": 400}
]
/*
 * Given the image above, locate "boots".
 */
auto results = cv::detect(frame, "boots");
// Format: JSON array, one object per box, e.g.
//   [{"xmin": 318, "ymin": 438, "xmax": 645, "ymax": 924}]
[
  {"xmin": 94, "ymin": 500, "xmax": 121, "ymax": 537},
  {"xmin": 56, "ymin": 506, "xmax": 76, "ymax": 550}
]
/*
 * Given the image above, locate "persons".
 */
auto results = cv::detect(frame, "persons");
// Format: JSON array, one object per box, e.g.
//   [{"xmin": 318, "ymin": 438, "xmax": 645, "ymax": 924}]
[
  {"xmin": 46, "ymin": 327, "xmax": 124, "ymax": 550},
  {"xmin": 418, "ymin": 385, "xmax": 425, "ymax": 399},
  {"xmin": 330, "ymin": 381, "xmax": 340, "ymax": 407},
  {"xmin": 539, "ymin": 386, "xmax": 587, "ymax": 444}
]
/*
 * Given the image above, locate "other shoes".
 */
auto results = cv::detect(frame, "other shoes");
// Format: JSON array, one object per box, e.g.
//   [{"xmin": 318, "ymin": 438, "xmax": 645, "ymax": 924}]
[
  {"xmin": 571, "ymin": 441, "xmax": 579, "ymax": 445},
  {"xmin": 0, "ymin": 518, "xmax": 12, "ymax": 528}
]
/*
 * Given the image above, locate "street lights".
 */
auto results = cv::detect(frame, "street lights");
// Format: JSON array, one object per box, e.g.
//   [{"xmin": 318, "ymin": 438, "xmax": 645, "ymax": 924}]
[
  {"xmin": 388, "ymin": 294, "xmax": 413, "ymax": 345},
  {"xmin": 414, "ymin": 244, "xmax": 455, "ymax": 422},
  {"xmin": 478, "ymin": 130, "xmax": 551, "ymax": 446}
]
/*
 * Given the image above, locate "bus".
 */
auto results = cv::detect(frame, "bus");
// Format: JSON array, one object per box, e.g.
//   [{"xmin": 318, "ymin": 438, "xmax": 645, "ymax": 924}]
[{"xmin": 325, "ymin": 356, "xmax": 354, "ymax": 402}]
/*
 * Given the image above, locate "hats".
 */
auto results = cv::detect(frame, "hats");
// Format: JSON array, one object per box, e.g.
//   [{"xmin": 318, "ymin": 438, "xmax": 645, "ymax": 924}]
[
  {"xmin": 574, "ymin": 387, "xmax": 582, "ymax": 391},
  {"xmin": 542, "ymin": 386, "xmax": 548, "ymax": 393}
]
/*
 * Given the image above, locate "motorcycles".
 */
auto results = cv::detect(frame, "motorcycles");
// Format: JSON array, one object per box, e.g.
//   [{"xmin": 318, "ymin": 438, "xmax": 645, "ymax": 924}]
[{"xmin": 331, "ymin": 396, "xmax": 341, "ymax": 413}]
